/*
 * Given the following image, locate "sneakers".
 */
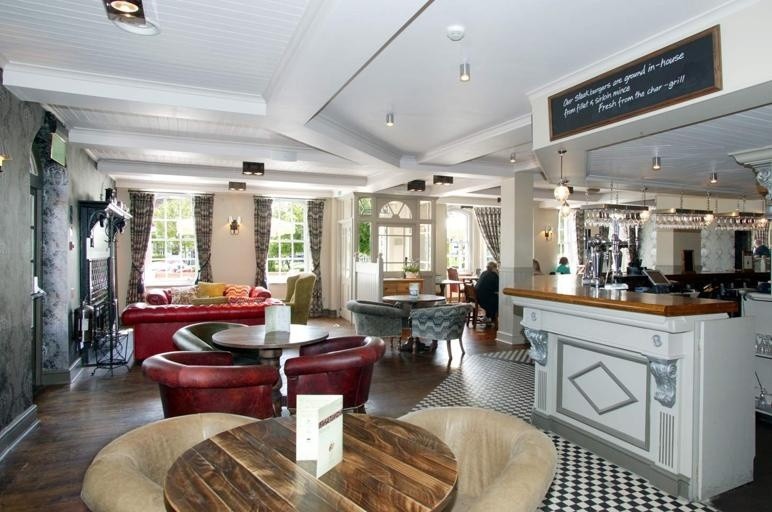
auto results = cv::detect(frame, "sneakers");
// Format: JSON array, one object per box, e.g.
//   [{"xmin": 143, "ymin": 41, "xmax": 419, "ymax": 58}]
[{"xmin": 480, "ymin": 316, "xmax": 491, "ymax": 322}]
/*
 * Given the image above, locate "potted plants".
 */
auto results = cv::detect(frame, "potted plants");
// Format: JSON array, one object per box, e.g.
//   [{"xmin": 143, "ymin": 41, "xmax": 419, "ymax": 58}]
[{"xmin": 402, "ymin": 257, "xmax": 420, "ymax": 279}]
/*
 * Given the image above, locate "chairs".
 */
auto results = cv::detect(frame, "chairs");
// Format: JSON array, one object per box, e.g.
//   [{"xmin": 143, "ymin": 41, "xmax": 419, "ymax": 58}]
[
  {"xmin": 283, "ymin": 332, "xmax": 386, "ymax": 416},
  {"xmin": 170, "ymin": 320, "xmax": 265, "ymax": 366},
  {"xmin": 140, "ymin": 348, "xmax": 282, "ymax": 420},
  {"xmin": 75, "ymin": 411, "xmax": 268, "ymax": 512},
  {"xmin": 280, "ymin": 272, "xmax": 318, "ymax": 326},
  {"xmin": 346, "ymin": 298, "xmax": 408, "ymax": 348},
  {"xmin": 92, "ymin": 302, "xmax": 131, "ymax": 375},
  {"xmin": 396, "ymin": 399, "xmax": 562, "ymax": 512},
  {"xmin": 409, "ymin": 303, "xmax": 474, "ymax": 370},
  {"xmin": 444, "ymin": 264, "xmax": 487, "ymax": 328}
]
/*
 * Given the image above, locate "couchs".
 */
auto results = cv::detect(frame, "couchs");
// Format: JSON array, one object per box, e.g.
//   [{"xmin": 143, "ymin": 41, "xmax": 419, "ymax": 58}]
[
  {"xmin": 145, "ymin": 281, "xmax": 273, "ymax": 306},
  {"xmin": 119, "ymin": 300, "xmax": 279, "ymax": 367}
]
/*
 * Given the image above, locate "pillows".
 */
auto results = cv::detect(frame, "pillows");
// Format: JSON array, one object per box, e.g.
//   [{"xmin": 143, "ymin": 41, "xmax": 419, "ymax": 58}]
[
  {"xmin": 169, "ymin": 285, "xmax": 200, "ymax": 305},
  {"xmin": 198, "ymin": 282, "xmax": 226, "ymax": 299},
  {"xmin": 190, "ymin": 296, "xmax": 228, "ymax": 304},
  {"xmin": 224, "ymin": 283, "xmax": 253, "ymax": 298},
  {"xmin": 228, "ymin": 295, "xmax": 265, "ymax": 302}
]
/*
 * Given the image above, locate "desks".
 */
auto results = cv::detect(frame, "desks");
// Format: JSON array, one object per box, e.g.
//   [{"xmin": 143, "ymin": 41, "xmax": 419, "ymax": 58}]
[
  {"xmin": 211, "ymin": 325, "xmax": 331, "ymax": 417},
  {"xmin": 381, "ymin": 293, "xmax": 449, "ymax": 353},
  {"xmin": 161, "ymin": 411, "xmax": 461, "ymax": 512},
  {"xmin": 435, "ymin": 280, "xmax": 465, "ymax": 295}
]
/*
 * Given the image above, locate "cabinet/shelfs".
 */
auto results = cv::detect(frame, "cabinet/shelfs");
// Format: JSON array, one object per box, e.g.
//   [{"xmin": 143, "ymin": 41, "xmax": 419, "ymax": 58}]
[
  {"xmin": 383, "ymin": 277, "xmax": 427, "ymax": 296},
  {"xmin": 738, "ymin": 287, "xmax": 772, "ymax": 416}
]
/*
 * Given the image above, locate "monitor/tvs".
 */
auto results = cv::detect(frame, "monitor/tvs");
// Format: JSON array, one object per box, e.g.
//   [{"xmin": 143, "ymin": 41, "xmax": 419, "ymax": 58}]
[{"xmin": 642, "ymin": 269, "xmax": 670, "ymax": 285}]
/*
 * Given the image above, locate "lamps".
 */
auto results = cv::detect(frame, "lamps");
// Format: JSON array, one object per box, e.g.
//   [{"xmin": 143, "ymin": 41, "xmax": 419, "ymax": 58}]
[
  {"xmin": 710, "ymin": 172, "xmax": 718, "ymax": 184},
  {"xmin": 103, "ymin": 0, "xmax": 162, "ymax": 38},
  {"xmin": 386, "ymin": 112, "xmax": 396, "ymax": 127},
  {"xmin": 229, "ymin": 182, "xmax": 246, "ymax": 191},
  {"xmin": 460, "ymin": 62, "xmax": 470, "ymax": 82},
  {"xmin": 552, "ymin": 148, "xmax": 571, "ymax": 202},
  {"xmin": 229, "ymin": 216, "xmax": 241, "ymax": 234},
  {"xmin": 652, "ymin": 156, "xmax": 661, "ymax": 170},
  {"xmin": 242, "ymin": 161, "xmax": 265, "ymax": 175},
  {"xmin": 544, "ymin": 223, "xmax": 552, "ymax": 241},
  {"xmin": 433, "ymin": 175, "xmax": 453, "ymax": 186},
  {"xmin": 407, "ymin": 179, "xmax": 426, "ymax": 193}
]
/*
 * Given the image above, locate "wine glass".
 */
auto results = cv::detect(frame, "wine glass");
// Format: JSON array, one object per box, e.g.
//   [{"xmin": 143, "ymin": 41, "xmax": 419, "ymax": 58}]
[{"xmin": 580, "ymin": 206, "xmax": 767, "ymax": 235}]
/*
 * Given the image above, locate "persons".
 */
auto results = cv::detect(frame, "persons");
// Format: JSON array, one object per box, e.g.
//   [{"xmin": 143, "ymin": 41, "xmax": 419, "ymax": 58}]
[
  {"xmin": 556, "ymin": 257, "xmax": 570, "ymax": 273},
  {"xmin": 477, "ymin": 262, "xmax": 499, "ymax": 328},
  {"xmin": 753, "ymin": 240, "xmax": 769, "ymax": 256},
  {"xmin": 479, "ymin": 312, "xmax": 492, "ymax": 323},
  {"xmin": 533, "ymin": 258, "xmax": 543, "ymax": 274}
]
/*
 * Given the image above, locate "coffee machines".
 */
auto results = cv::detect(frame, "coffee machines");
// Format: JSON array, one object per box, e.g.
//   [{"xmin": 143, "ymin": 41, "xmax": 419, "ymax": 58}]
[{"xmin": 577, "ymin": 232, "xmax": 623, "ymax": 287}]
